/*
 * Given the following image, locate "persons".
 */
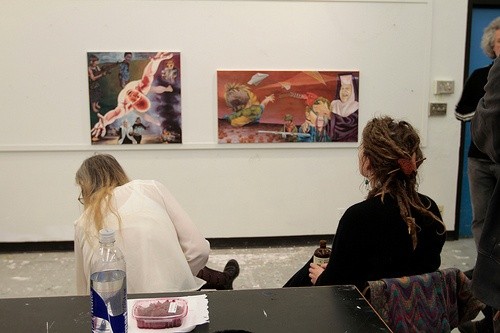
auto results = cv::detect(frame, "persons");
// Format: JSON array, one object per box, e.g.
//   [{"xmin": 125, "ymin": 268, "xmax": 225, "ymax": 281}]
[
  {"xmin": 74, "ymin": 154, "xmax": 240, "ymax": 296},
  {"xmin": 281, "ymin": 115, "xmax": 446, "ymax": 292},
  {"xmin": 454, "ymin": 16, "xmax": 500, "ymax": 306}
]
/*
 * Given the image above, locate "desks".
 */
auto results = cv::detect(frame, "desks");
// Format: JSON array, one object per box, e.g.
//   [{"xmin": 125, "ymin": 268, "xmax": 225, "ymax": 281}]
[{"xmin": 0, "ymin": 284, "xmax": 390, "ymax": 333}]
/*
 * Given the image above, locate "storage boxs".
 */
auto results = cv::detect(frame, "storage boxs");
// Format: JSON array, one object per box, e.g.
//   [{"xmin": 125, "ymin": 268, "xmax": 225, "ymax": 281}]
[{"xmin": 132, "ymin": 299, "xmax": 189, "ymax": 330}]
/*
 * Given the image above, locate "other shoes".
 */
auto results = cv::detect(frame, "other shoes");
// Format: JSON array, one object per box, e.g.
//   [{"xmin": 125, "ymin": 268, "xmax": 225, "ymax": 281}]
[{"xmin": 224, "ymin": 259, "xmax": 241, "ymax": 292}]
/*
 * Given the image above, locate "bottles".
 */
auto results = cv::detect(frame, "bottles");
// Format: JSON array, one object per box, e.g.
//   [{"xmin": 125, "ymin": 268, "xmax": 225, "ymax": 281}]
[
  {"xmin": 314, "ymin": 240, "xmax": 331, "ymax": 268},
  {"xmin": 90, "ymin": 230, "xmax": 128, "ymax": 333}
]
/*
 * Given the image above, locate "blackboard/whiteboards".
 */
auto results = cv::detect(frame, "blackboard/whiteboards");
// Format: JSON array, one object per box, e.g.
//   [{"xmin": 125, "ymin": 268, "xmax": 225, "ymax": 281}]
[{"xmin": 0, "ymin": 1, "xmax": 428, "ymax": 152}]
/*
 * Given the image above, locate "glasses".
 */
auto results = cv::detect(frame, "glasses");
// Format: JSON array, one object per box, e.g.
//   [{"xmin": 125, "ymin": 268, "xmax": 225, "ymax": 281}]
[{"xmin": 78, "ymin": 190, "xmax": 86, "ymax": 204}]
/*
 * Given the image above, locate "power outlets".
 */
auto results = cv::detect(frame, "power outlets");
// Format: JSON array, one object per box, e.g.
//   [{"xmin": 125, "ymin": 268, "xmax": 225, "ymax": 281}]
[
  {"xmin": 429, "ymin": 103, "xmax": 447, "ymax": 116},
  {"xmin": 435, "ymin": 80, "xmax": 455, "ymax": 94}
]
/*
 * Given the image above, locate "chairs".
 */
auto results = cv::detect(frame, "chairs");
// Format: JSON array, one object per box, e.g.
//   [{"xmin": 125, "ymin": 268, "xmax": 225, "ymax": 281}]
[{"xmin": 363, "ymin": 268, "xmax": 461, "ymax": 333}]
